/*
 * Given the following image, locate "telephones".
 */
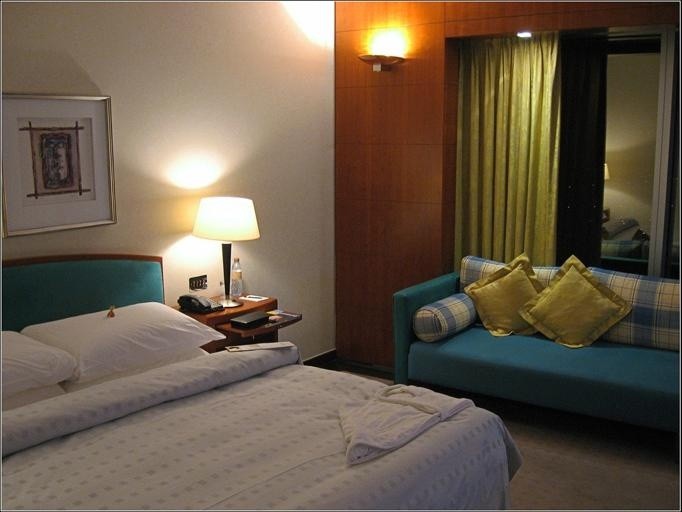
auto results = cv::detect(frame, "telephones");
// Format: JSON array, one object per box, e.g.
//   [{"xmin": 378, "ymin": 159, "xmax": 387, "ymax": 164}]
[{"xmin": 177, "ymin": 295, "xmax": 224, "ymax": 314}]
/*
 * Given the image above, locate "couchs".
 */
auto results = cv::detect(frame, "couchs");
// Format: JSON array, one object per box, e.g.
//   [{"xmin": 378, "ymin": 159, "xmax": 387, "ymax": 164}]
[{"xmin": 393, "ymin": 256, "xmax": 679, "ymax": 432}]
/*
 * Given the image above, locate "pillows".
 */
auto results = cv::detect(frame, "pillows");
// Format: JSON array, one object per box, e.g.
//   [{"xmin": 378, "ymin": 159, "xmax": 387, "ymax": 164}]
[
  {"xmin": 63, "ymin": 349, "xmax": 210, "ymax": 391},
  {"xmin": 412, "ymin": 292, "xmax": 477, "ymax": 342},
  {"xmin": 642, "ymin": 238, "xmax": 680, "ymax": 264},
  {"xmin": 459, "ymin": 256, "xmax": 562, "ymax": 294},
  {"xmin": 517, "ymin": 254, "xmax": 633, "ymax": 349},
  {"xmin": 1, "ymin": 331, "xmax": 77, "ymax": 397},
  {"xmin": 464, "ymin": 252, "xmax": 545, "ymax": 337},
  {"xmin": 19, "ymin": 302, "xmax": 227, "ymax": 381},
  {"xmin": 2, "ymin": 386, "xmax": 67, "ymax": 410},
  {"xmin": 600, "ymin": 239, "xmax": 641, "ymax": 260},
  {"xmin": 588, "ymin": 264, "xmax": 679, "ymax": 351}
]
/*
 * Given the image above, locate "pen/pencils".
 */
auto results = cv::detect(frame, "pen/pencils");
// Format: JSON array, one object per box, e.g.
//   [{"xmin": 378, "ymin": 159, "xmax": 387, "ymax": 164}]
[{"xmin": 244, "ymin": 296, "xmax": 262, "ymax": 299}]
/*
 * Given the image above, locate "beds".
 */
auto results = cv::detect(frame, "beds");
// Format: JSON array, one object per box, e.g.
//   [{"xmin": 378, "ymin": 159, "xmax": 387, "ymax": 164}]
[{"xmin": 1, "ymin": 254, "xmax": 523, "ymax": 511}]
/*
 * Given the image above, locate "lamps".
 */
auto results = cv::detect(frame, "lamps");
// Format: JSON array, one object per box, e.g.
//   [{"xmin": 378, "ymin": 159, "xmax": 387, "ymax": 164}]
[
  {"xmin": 358, "ymin": 32, "xmax": 406, "ymax": 71},
  {"xmin": 605, "ymin": 163, "xmax": 610, "ymax": 180},
  {"xmin": 193, "ymin": 196, "xmax": 261, "ymax": 309}
]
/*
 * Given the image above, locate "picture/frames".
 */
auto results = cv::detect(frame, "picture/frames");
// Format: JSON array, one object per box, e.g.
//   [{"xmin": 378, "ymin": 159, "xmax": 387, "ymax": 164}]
[{"xmin": 1, "ymin": 91, "xmax": 118, "ymax": 238}]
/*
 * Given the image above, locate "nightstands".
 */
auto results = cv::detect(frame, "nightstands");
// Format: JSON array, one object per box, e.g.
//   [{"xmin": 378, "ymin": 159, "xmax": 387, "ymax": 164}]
[
  {"xmin": 174, "ymin": 293, "xmax": 303, "ymax": 353},
  {"xmin": 603, "ymin": 207, "xmax": 610, "ymax": 223}
]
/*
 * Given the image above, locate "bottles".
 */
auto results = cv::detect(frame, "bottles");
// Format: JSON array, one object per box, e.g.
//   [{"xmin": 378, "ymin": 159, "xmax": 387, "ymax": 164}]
[{"xmin": 230, "ymin": 258, "xmax": 242, "ymax": 297}]
